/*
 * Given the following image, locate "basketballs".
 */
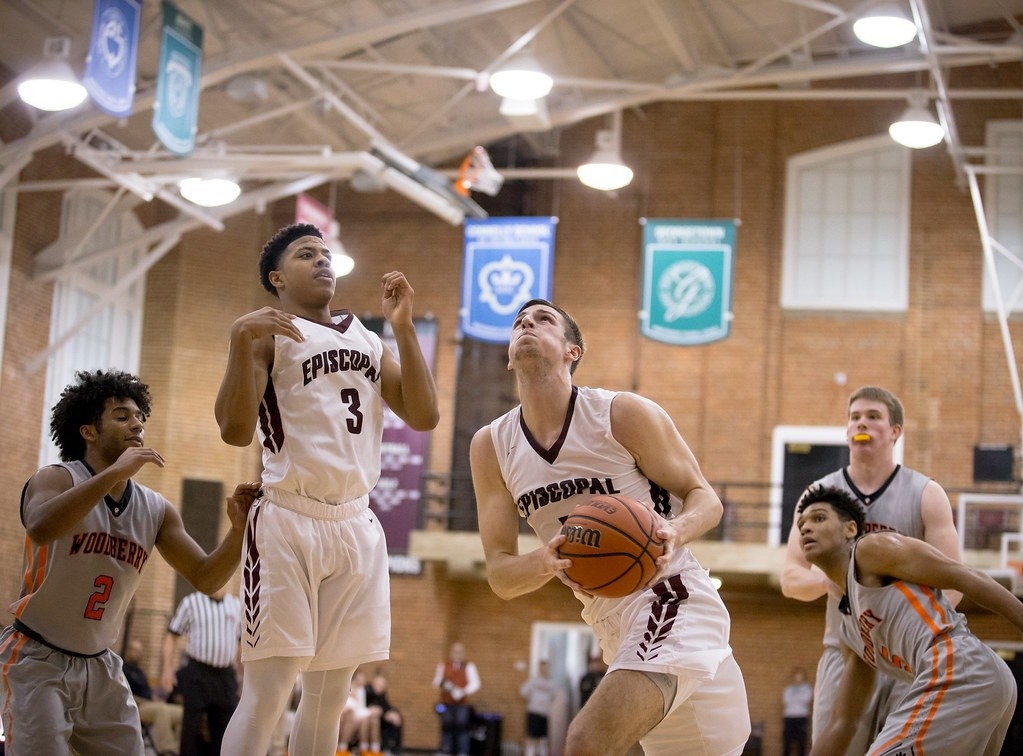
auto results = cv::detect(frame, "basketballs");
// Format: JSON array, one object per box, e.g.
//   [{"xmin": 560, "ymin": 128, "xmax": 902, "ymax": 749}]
[{"xmin": 558, "ymin": 496, "xmax": 664, "ymax": 598}]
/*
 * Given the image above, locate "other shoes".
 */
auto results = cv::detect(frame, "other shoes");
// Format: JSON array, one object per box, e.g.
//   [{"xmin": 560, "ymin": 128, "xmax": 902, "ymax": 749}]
[
  {"xmin": 335, "ymin": 748, "xmax": 351, "ymax": 756},
  {"xmin": 360, "ymin": 750, "xmax": 384, "ymax": 756}
]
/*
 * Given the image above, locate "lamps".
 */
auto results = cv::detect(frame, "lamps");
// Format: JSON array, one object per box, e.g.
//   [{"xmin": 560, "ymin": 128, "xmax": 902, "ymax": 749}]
[
  {"xmin": 15, "ymin": 35, "xmax": 87, "ymax": 112},
  {"xmin": 889, "ymin": 70, "xmax": 944, "ymax": 150},
  {"xmin": 853, "ymin": 0, "xmax": 917, "ymax": 49},
  {"xmin": 180, "ymin": 138, "xmax": 241, "ymax": 208},
  {"xmin": 577, "ymin": 113, "xmax": 633, "ymax": 191},
  {"xmin": 490, "ymin": 38, "xmax": 554, "ymax": 101},
  {"xmin": 325, "ymin": 179, "xmax": 354, "ymax": 278}
]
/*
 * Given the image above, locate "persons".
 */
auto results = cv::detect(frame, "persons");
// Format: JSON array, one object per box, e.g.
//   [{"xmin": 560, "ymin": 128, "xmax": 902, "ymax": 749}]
[
  {"xmin": 214, "ymin": 224, "xmax": 440, "ymax": 756},
  {"xmin": 469, "ymin": 299, "xmax": 753, "ymax": 756},
  {"xmin": 120, "ymin": 637, "xmax": 184, "ymax": 756},
  {"xmin": 337, "ymin": 667, "xmax": 404, "ymax": 755},
  {"xmin": 780, "ymin": 385, "xmax": 963, "ymax": 756},
  {"xmin": 798, "ymin": 484, "xmax": 1023, "ymax": 756},
  {"xmin": 1, "ymin": 367, "xmax": 263, "ymax": 756},
  {"xmin": 781, "ymin": 665, "xmax": 814, "ymax": 755},
  {"xmin": 162, "ymin": 585, "xmax": 242, "ymax": 756},
  {"xmin": 432, "ymin": 641, "xmax": 482, "ymax": 756},
  {"xmin": 580, "ymin": 646, "xmax": 609, "ymax": 710},
  {"xmin": 519, "ymin": 658, "xmax": 560, "ymax": 756}
]
